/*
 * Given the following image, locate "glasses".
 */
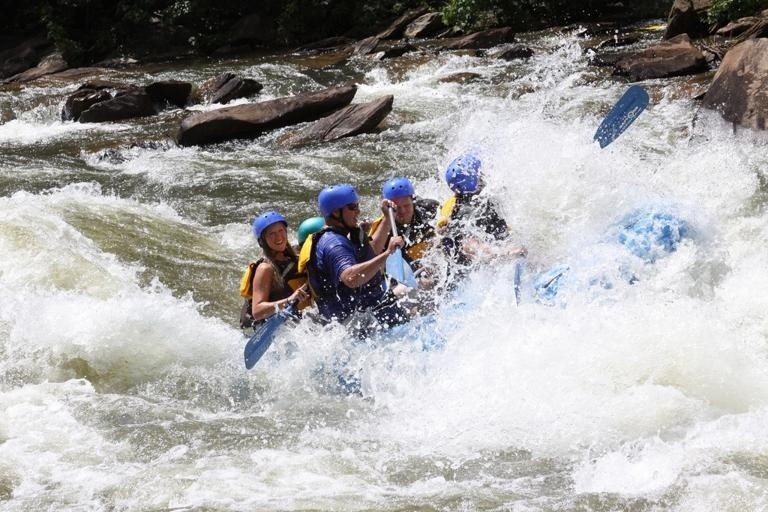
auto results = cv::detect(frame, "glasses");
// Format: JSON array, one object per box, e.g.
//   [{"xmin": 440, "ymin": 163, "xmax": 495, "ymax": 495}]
[{"xmin": 345, "ymin": 202, "xmax": 359, "ymax": 211}]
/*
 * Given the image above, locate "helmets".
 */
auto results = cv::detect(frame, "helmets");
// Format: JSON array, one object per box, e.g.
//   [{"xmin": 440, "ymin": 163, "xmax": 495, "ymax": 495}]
[
  {"xmin": 318, "ymin": 183, "xmax": 359, "ymax": 218},
  {"xmin": 382, "ymin": 177, "xmax": 414, "ymax": 199},
  {"xmin": 298, "ymin": 217, "xmax": 326, "ymax": 244},
  {"xmin": 253, "ymin": 212, "xmax": 287, "ymax": 237},
  {"xmin": 448, "ymin": 155, "xmax": 481, "ymax": 195}
]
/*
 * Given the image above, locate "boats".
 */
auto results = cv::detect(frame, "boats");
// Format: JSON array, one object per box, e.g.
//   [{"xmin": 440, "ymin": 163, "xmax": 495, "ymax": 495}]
[{"xmin": 239, "ymin": 208, "xmax": 707, "ymax": 415}]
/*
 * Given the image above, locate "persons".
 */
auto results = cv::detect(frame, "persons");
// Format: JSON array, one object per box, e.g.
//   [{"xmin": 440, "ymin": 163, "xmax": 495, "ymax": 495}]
[
  {"xmin": 370, "ymin": 177, "xmax": 443, "ymax": 278},
  {"xmin": 290, "ymin": 217, "xmax": 326, "ymax": 257},
  {"xmin": 437, "ymin": 155, "xmax": 528, "ymax": 270},
  {"xmin": 241, "ymin": 212, "xmax": 313, "ymax": 321},
  {"xmin": 298, "ymin": 184, "xmax": 432, "ymax": 341}
]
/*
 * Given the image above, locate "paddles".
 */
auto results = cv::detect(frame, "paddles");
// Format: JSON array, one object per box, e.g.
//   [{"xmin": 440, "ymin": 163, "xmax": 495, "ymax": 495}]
[
  {"xmin": 514, "ymin": 255, "xmax": 526, "ymax": 307},
  {"xmin": 385, "ymin": 206, "xmax": 416, "ymax": 285},
  {"xmin": 594, "ymin": 84, "xmax": 648, "ymax": 150},
  {"xmin": 244, "ymin": 287, "xmax": 308, "ymax": 371}
]
